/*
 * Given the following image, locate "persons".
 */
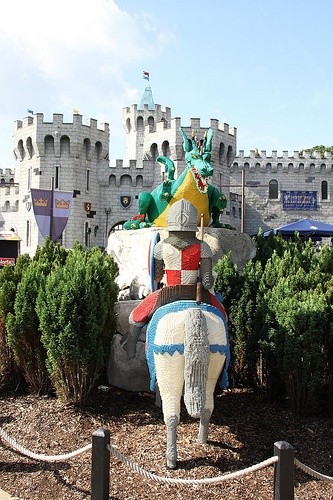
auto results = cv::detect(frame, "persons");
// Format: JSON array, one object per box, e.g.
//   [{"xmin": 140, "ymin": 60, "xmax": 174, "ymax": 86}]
[{"xmin": 122, "ymin": 198, "xmax": 227, "ymax": 362}]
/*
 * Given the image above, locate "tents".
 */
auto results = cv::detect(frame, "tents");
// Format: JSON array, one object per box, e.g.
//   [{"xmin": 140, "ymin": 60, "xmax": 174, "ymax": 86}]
[{"xmin": 260, "ymin": 217, "xmax": 333, "ymax": 238}]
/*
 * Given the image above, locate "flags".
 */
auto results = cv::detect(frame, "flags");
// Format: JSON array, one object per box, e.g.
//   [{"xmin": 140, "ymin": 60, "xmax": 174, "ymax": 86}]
[
  {"xmin": 73, "ymin": 108, "xmax": 78, "ymax": 113},
  {"xmin": 26, "ymin": 109, "xmax": 32, "ymax": 113},
  {"xmin": 143, "ymin": 70, "xmax": 149, "ymax": 81}
]
